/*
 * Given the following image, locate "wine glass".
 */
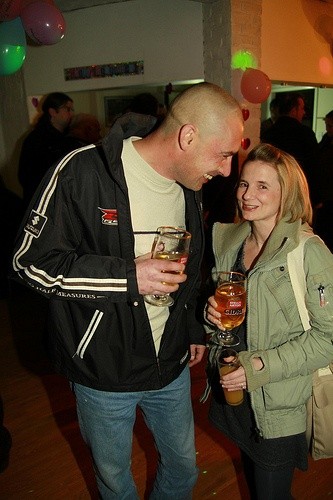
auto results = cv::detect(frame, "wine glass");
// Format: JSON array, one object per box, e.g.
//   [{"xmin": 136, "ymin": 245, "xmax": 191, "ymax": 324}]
[
  {"xmin": 144, "ymin": 226, "xmax": 192, "ymax": 307},
  {"xmin": 210, "ymin": 272, "xmax": 247, "ymax": 346}
]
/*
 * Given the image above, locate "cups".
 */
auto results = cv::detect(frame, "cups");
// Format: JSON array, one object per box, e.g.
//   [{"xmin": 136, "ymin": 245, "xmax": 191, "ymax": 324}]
[{"xmin": 216, "ymin": 349, "xmax": 244, "ymax": 405}]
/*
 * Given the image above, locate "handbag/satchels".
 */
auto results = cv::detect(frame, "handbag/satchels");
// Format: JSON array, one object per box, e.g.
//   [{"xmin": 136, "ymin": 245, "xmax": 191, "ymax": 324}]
[{"xmin": 305, "ymin": 360, "xmax": 333, "ymax": 461}]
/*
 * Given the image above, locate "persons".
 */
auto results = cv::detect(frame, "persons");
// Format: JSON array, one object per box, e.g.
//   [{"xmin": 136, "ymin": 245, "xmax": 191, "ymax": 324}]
[
  {"xmin": 11, "ymin": 82, "xmax": 246, "ymax": 500},
  {"xmin": 47, "ymin": 114, "xmax": 102, "ymax": 166},
  {"xmin": 270, "ymin": 95, "xmax": 323, "ymax": 236},
  {"xmin": 18, "ymin": 92, "xmax": 90, "ymax": 204},
  {"xmin": 109, "ymin": 92, "xmax": 158, "ymax": 129},
  {"xmin": 261, "ymin": 98, "xmax": 280, "ymax": 142},
  {"xmin": 202, "ymin": 171, "xmax": 239, "ymax": 281},
  {"xmin": 202, "ymin": 142, "xmax": 332, "ymax": 500},
  {"xmin": 159, "ymin": 103, "xmax": 164, "ymax": 114},
  {"xmin": 320, "ymin": 109, "xmax": 333, "ymax": 253}
]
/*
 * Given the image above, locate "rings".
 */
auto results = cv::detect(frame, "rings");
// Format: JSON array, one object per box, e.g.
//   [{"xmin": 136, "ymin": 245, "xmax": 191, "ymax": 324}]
[{"xmin": 241, "ymin": 383, "xmax": 246, "ymax": 389}]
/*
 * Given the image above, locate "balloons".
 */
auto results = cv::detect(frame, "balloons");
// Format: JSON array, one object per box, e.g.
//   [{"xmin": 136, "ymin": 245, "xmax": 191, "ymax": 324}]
[
  {"xmin": 0, "ymin": 0, "xmax": 65, "ymax": 77},
  {"xmin": 230, "ymin": 48, "xmax": 272, "ymax": 105}
]
